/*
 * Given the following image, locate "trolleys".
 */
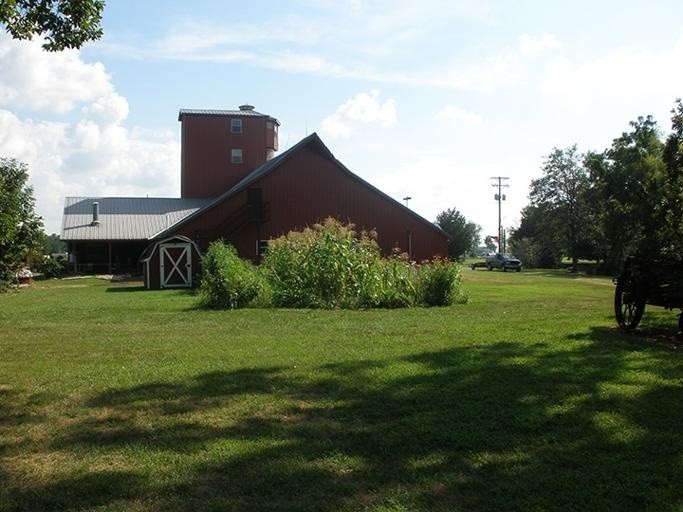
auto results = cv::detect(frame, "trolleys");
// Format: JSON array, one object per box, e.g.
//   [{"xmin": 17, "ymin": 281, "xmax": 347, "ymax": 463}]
[{"xmin": 611, "ymin": 247, "xmax": 683, "ymax": 333}]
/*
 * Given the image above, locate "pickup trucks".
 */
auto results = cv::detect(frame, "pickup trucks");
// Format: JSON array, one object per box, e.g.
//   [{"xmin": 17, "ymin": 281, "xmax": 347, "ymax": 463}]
[{"xmin": 485, "ymin": 252, "xmax": 522, "ymax": 271}]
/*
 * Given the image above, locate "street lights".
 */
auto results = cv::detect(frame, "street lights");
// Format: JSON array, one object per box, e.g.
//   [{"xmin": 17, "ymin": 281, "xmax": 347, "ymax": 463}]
[
  {"xmin": 402, "ymin": 196, "xmax": 413, "ymax": 210},
  {"xmin": 494, "ymin": 193, "xmax": 505, "ymax": 253}
]
